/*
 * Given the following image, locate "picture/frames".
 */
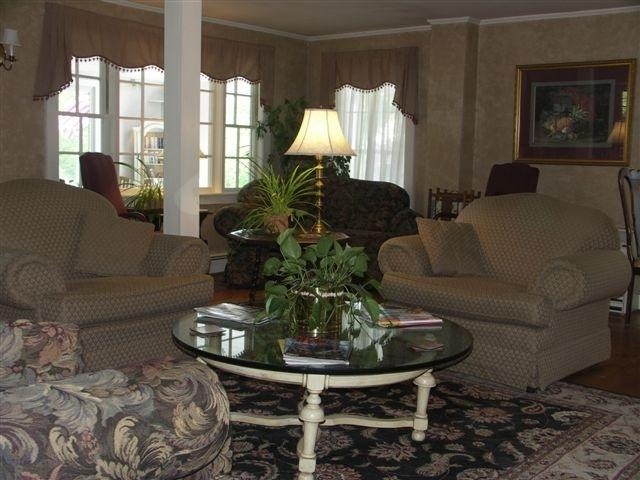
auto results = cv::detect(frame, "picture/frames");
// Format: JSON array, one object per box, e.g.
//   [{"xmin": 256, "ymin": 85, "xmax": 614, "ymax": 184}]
[{"xmin": 513, "ymin": 58, "xmax": 636, "ymax": 167}]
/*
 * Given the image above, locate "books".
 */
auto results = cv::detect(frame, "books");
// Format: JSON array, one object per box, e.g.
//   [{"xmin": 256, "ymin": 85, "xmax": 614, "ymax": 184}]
[
  {"xmin": 278, "ymin": 336, "xmax": 352, "ymax": 365},
  {"xmin": 194, "ymin": 303, "xmax": 278, "ymax": 324}
]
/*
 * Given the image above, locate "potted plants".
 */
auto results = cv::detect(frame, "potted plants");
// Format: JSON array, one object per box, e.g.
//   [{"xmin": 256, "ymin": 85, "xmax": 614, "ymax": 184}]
[
  {"xmin": 253, "ymin": 228, "xmax": 393, "ymax": 340},
  {"xmin": 240, "ymin": 156, "xmax": 331, "ymax": 236}
]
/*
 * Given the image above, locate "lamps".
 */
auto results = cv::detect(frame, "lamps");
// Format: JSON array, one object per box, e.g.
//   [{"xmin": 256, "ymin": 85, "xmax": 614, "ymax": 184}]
[
  {"xmin": 0, "ymin": 28, "xmax": 22, "ymax": 71},
  {"xmin": 284, "ymin": 109, "xmax": 357, "ymax": 234}
]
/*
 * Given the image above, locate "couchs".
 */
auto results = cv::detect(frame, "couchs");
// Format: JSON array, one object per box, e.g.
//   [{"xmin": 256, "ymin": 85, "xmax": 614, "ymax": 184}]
[
  {"xmin": 0, "ymin": 319, "xmax": 230, "ymax": 480},
  {"xmin": 213, "ymin": 177, "xmax": 423, "ymax": 290},
  {"xmin": 378, "ymin": 193, "xmax": 631, "ymax": 392},
  {"xmin": 0, "ymin": 178, "xmax": 213, "ymax": 370}
]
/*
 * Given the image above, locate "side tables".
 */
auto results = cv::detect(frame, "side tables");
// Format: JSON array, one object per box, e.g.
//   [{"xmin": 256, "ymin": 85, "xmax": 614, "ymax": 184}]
[{"xmin": 126, "ymin": 208, "xmax": 215, "ymax": 245}]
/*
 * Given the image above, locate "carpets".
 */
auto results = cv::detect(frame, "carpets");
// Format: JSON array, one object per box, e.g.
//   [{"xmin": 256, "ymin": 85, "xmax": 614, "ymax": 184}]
[{"xmin": 213, "ymin": 369, "xmax": 640, "ymax": 480}]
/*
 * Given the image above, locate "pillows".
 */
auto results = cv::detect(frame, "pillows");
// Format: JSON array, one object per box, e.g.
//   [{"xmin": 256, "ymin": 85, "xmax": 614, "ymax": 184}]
[
  {"xmin": 74, "ymin": 210, "xmax": 155, "ymax": 277},
  {"xmin": 416, "ymin": 217, "xmax": 488, "ymax": 276}
]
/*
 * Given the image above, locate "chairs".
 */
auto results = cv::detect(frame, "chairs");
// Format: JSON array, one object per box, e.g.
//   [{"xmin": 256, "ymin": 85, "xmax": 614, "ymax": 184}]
[
  {"xmin": 618, "ymin": 168, "xmax": 640, "ymax": 329},
  {"xmin": 484, "ymin": 161, "xmax": 540, "ymax": 196},
  {"xmin": 79, "ymin": 152, "xmax": 146, "ymax": 222}
]
